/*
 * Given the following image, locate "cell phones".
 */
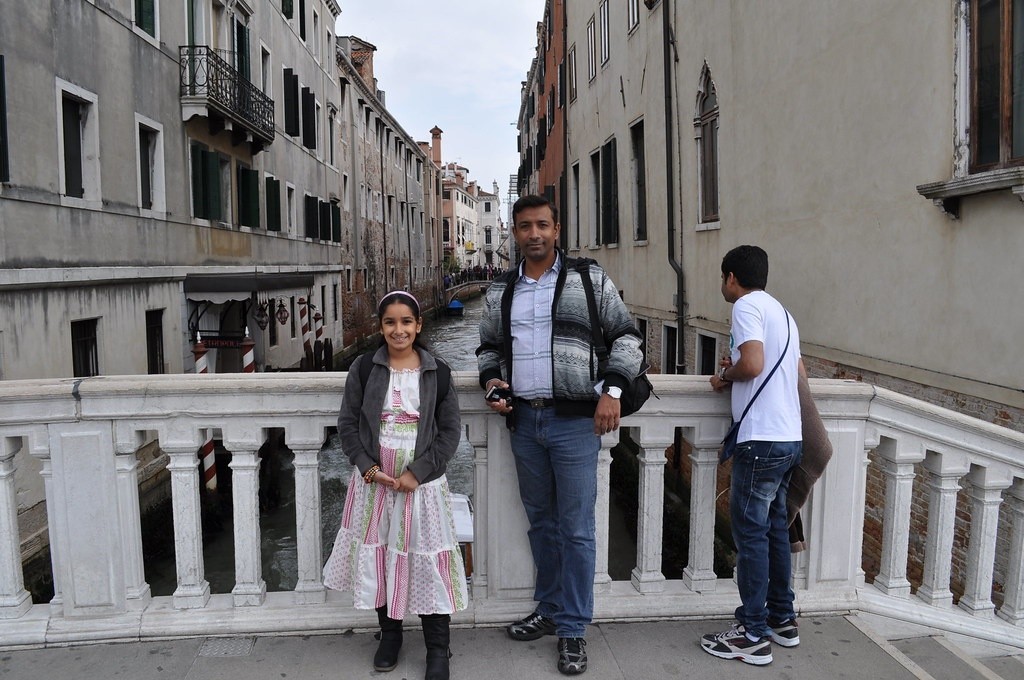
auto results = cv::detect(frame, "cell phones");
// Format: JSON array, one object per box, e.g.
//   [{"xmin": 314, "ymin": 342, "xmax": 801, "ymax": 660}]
[{"xmin": 486, "ymin": 385, "xmax": 513, "ymax": 406}]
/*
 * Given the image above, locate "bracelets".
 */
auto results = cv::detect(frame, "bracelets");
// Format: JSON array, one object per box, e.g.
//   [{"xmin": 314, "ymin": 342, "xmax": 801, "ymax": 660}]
[{"xmin": 364, "ymin": 464, "xmax": 380, "ymax": 485}]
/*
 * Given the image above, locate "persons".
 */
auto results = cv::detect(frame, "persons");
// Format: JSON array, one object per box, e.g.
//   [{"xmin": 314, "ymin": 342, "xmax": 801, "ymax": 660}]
[
  {"xmin": 474, "ymin": 195, "xmax": 644, "ymax": 674},
  {"xmin": 700, "ymin": 245, "xmax": 810, "ymax": 667},
  {"xmin": 321, "ymin": 290, "xmax": 468, "ymax": 679},
  {"xmin": 445, "ymin": 264, "xmax": 507, "ymax": 289}
]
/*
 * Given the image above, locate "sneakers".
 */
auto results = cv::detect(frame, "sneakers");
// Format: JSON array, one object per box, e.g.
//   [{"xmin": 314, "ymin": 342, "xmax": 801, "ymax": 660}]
[
  {"xmin": 506, "ymin": 614, "xmax": 556, "ymax": 642},
  {"xmin": 558, "ymin": 636, "xmax": 588, "ymax": 675},
  {"xmin": 701, "ymin": 619, "xmax": 800, "ymax": 667}
]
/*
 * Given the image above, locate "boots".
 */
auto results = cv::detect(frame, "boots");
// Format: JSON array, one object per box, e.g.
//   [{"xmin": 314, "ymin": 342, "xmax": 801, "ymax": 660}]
[
  {"xmin": 420, "ymin": 614, "xmax": 453, "ymax": 680},
  {"xmin": 373, "ymin": 606, "xmax": 403, "ymax": 672}
]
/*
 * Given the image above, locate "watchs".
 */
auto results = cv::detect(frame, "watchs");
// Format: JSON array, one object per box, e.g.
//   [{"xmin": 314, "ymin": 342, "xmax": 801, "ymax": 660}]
[
  {"xmin": 602, "ymin": 385, "xmax": 624, "ymax": 399},
  {"xmin": 718, "ymin": 367, "xmax": 732, "ymax": 383}
]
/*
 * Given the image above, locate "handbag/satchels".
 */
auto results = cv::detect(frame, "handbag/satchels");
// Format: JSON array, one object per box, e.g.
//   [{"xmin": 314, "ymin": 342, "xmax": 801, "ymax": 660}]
[
  {"xmin": 720, "ymin": 422, "xmax": 741, "ymax": 461},
  {"xmin": 600, "ymin": 361, "xmax": 652, "ymax": 415}
]
